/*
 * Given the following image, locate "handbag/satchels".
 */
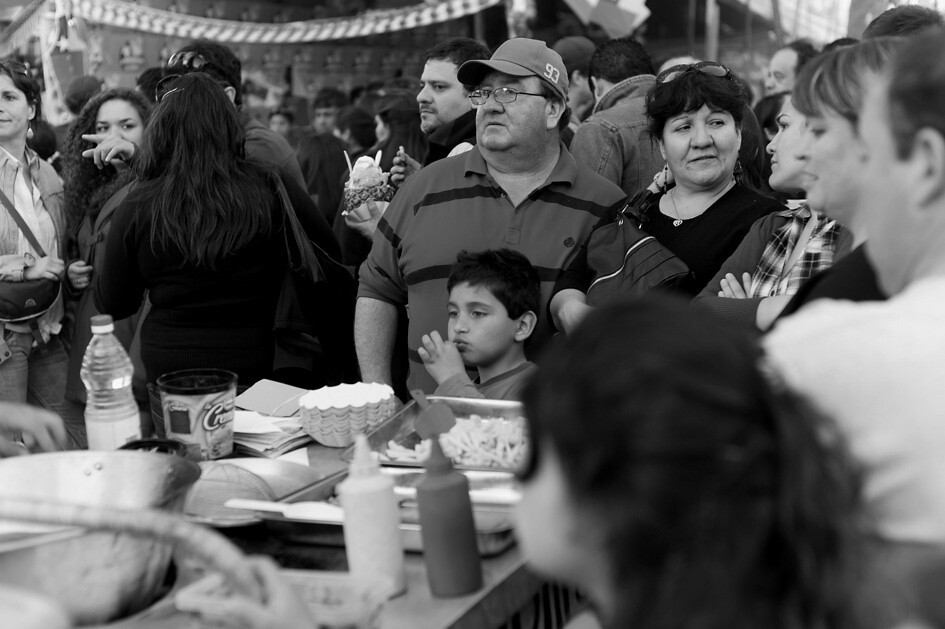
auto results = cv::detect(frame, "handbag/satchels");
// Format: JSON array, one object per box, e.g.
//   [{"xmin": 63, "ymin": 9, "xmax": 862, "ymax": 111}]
[{"xmin": 2, "ymin": 274, "xmax": 62, "ymax": 324}]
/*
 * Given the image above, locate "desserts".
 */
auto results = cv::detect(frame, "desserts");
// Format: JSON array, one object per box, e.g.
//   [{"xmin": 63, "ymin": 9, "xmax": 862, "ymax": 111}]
[{"xmin": 343, "ymin": 150, "xmax": 394, "ymax": 212}]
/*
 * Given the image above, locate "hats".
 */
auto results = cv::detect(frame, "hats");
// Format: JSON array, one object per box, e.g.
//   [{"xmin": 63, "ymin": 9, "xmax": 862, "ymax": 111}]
[{"xmin": 457, "ymin": 36, "xmax": 569, "ymax": 100}]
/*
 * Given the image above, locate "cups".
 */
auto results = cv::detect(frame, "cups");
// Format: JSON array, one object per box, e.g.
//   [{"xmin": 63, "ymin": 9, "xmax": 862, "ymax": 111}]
[{"xmin": 157, "ymin": 369, "xmax": 237, "ymax": 460}]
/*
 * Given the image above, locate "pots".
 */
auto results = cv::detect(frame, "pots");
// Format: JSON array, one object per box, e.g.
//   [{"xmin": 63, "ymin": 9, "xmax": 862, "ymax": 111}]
[{"xmin": 0, "ymin": 438, "xmax": 202, "ymax": 629}]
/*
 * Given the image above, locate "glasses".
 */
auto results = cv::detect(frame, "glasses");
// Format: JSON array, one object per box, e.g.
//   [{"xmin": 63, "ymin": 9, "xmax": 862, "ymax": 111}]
[
  {"xmin": 6, "ymin": 57, "xmax": 31, "ymax": 80},
  {"xmin": 467, "ymin": 87, "xmax": 546, "ymax": 106},
  {"xmin": 165, "ymin": 50, "xmax": 235, "ymax": 84},
  {"xmin": 656, "ymin": 60, "xmax": 735, "ymax": 84},
  {"xmin": 155, "ymin": 73, "xmax": 210, "ymax": 105}
]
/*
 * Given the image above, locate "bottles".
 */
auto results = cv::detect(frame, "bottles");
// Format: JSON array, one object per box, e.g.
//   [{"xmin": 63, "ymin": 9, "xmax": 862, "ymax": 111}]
[
  {"xmin": 417, "ymin": 439, "xmax": 483, "ymax": 598},
  {"xmin": 81, "ymin": 315, "xmax": 142, "ymax": 450},
  {"xmin": 338, "ymin": 434, "xmax": 407, "ymax": 598}
]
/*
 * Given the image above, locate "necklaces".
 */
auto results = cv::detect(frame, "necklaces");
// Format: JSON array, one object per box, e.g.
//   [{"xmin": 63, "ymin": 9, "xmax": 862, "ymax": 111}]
[{"xmin": 670, "ymin": 176, "xmax": 735, "ymax": 228}]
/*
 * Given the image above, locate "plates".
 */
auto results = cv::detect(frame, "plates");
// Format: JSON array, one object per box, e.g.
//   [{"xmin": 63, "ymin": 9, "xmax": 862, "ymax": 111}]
[{"xmin": 299, "ymin": 382, "xmax": 396, "ymax": 448}]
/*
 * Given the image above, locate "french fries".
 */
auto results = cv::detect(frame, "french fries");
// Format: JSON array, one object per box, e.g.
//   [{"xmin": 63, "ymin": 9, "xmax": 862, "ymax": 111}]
[{"xmin": 380, "ymin": 414, "xmax": 527, "ymax": 466}]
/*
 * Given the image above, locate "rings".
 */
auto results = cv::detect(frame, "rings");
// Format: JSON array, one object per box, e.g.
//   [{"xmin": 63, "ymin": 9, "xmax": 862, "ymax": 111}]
[{"xmin": 77, "ymin": 278, "xmax": 79, "ymax": 283}]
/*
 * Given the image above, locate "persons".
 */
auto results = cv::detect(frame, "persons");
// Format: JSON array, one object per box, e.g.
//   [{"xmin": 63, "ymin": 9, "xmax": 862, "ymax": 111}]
[{"xmin": 0, "ymin": 1, "xmax": 944, "ymax": 628}]
